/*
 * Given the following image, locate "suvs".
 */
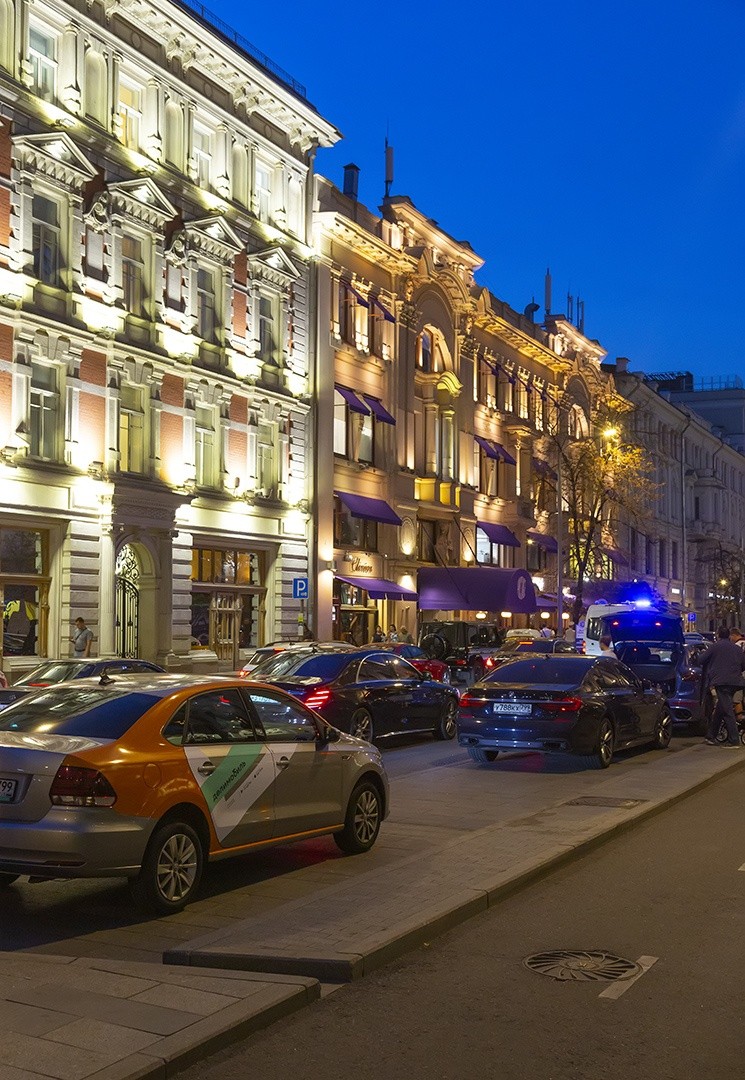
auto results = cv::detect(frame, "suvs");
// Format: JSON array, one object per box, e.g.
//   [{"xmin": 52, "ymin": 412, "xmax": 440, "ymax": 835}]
[
  {"xmin": 239, "ymin": 640, "xmax": 359, "ymax": 678},
  {"xmin": 416, "ymin": 620, "xmax": 505, "ymax": 670}
]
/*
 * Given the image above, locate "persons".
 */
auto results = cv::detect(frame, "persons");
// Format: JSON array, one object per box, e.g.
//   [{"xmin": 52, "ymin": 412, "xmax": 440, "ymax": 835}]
[
  {"xmin": 303, "ymin": 623, "xmax": 316, "ymax": 641},
  {"xmin": 370, "ymin": 624, "xmax": 415, "ymax": 644},
  {"xmin": 541, "ymin": 624, "xmax": 557, "ymax": 638},
  {"xmin": 69, "ymin": 617, "xmax": 93, "ymax": 657},
  {"xmin": 565, "ymin": 625, "xmax": 575, "ymax": 642},
  {"xmin": 599, "ymin": 636, "xmax": 617, "ymax": 659},
  {"xmin": 697, "ymin": 625, "xmax": 745, "ymax": 749}
]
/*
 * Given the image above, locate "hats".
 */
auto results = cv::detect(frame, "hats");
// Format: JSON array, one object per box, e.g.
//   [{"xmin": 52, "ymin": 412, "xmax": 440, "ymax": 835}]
[
  {"xmin": 399, "ymin": 625, "xmax": 408, "ymax": 634},
  {"xmin": 568, "ymin": 621, "xmax": 574, "ymax": 626}
]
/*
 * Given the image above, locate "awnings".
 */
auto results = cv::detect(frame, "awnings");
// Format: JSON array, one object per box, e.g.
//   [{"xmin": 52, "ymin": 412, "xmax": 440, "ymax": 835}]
[
  {"xmin": 335, "ymin": 576, "xmax": 418, "ymax": 601},
  {"xmin": 527, "ymin": 531, "xmax": 567, "ymax": 555},
  {"xmin": 481, "ymin": 356, "xmax": 560, "ymax": 409},
  {"xmin": 476, "ymin": 520, "xmax": 521, "ymax": 547},
  {"xmin": 574, "ymin": 580, "xmax": 652, "ymax": 604},
  {"xmin": 333, "ymin": 490, "xmax": 402, "ymax": 526},
  {"xmin": 542, "ymin": 594, "xmax": 593, "ymax": 612},
  {"xmin": 416, "ymin": 567, "xmax": 537, "ymax": 613},
  {"xmin": 656, "ymin": 602, "xmax": 702, "ymax": 615},
  {"xmin": 335, "ymin": 386, "xmax": 371, "ymax": 417},
  {"xmin": 475, "ymin": 435, "xmax": 516, "ymax": 466},
  {"xmin": 340, "ymin": 282, "xmax": 370, "ymax": 309},
  {"xmin": 368, "ymin": 297, "xmax": 397, "ymax": 324},
  {"xmin": 533, "ymin": 456, "xmax": 558, "ymax": 480},
  {"xmin": 363, "ymin": 396, "xmax": 396, "ymax": 426},
  {"xmin": 599, "ymin": 547, "xmax": 630, "ymax": 567},
  {"xmin": 535, "ymin": 598, "xmax": 557, "ymax": 612}
]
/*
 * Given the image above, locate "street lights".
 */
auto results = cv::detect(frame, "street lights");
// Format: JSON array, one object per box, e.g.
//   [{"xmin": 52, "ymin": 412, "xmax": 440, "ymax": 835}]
[{"xmin": 558, "ymin": 428, "xmax": 618, "ymax": 639}]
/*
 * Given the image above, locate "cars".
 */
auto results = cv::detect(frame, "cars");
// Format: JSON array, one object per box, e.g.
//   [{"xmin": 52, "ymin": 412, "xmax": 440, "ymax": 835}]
[
  {"xmin": 359, "ymin": 641, "xmax": 452, "ymax": 685},
  {"xmin": 461, "ymin": 596, "xmax": 724, "ymax": 731},
  {"xmin": 461, "ymin": 656, "xmax": 674, "ymax": 768},
  {"xmin": 238, "ymin": 647, "xmax": 462, "ymax": 749},
  {"xmin": 0, "ymin": 672, "xmax": 391, "ymax": 917},
  {"xmin": 1, "ymin": 656, "xmax": 167, "ymax": 714}
]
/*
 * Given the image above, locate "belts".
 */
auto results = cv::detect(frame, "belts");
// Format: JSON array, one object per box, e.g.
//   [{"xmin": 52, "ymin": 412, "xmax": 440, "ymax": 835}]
[{"xmin": 75, "ymin": 648, "xmax": 85, "ymax": 652}]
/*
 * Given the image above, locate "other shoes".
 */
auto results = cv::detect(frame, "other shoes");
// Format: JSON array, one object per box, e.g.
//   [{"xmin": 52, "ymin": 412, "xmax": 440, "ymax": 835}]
[
  {"xmin": 705, "ymin": 737, "xmax": 715, "ymax": 744},
  {"xmin": 720, "ymin": 742, "xmax": 741, "ymax": 749}
]
